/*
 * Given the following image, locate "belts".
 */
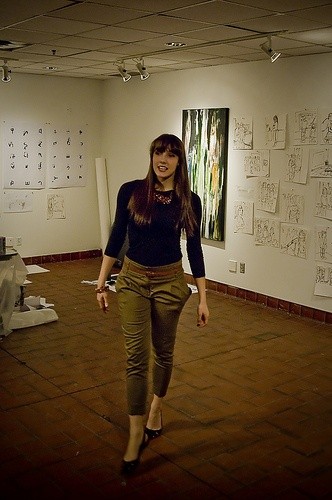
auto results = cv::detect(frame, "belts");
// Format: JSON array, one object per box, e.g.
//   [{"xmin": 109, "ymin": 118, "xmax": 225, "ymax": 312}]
[{"xmin": 123, "ymin": 260, "xmax": 182, "ymax": 278}]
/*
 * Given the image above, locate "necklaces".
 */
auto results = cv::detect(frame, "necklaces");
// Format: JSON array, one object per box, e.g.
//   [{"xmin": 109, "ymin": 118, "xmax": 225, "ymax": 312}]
[{"xmin": 154, "ymin": 191, "xmax": 172, "ymax": 204}]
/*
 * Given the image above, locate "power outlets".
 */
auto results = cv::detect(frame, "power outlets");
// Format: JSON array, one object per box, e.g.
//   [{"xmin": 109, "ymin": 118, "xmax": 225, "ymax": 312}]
[{"xmin": 239, "ymin": 263, "xmax": 246, "ymax": 273}]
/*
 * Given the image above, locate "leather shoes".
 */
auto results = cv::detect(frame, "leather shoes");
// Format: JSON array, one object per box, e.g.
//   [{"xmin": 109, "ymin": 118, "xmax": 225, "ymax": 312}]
[
  {"xmin": 145, "ymin": 409, "xmax": 163, "ymax": 439},
  {"xmin": 120, "ymin": 431, "xmax": 149, "ymax": 476}
]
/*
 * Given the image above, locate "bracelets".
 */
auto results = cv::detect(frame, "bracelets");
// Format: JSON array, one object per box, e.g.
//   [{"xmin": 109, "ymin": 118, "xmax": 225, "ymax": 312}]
[{"xmin": 96, "ymin": 286, "xmax": 108, "ymax": 294}]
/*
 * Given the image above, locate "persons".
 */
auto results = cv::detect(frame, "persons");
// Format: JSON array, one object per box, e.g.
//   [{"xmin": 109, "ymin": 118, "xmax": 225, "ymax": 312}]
[{"xmin": 94, "ymin": 134, "xmax": 210, "ymax": 475}]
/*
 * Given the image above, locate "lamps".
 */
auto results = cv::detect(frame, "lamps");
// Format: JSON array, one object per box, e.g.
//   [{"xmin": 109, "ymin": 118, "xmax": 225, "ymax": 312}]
[
  {"xmin": 1, "ymin": 59, "xmax": 12, "ymax": 83},
  {"xmin": 258, "ymin": 34, "xmax": 282, "ymax": 64},
  {"xmin": 132, "ymin": 56, "xmax": 151, "ymax": 80},
  {"xmin": 112, "ymin": 60, "xmax": 132, "ymax": 84}
]
